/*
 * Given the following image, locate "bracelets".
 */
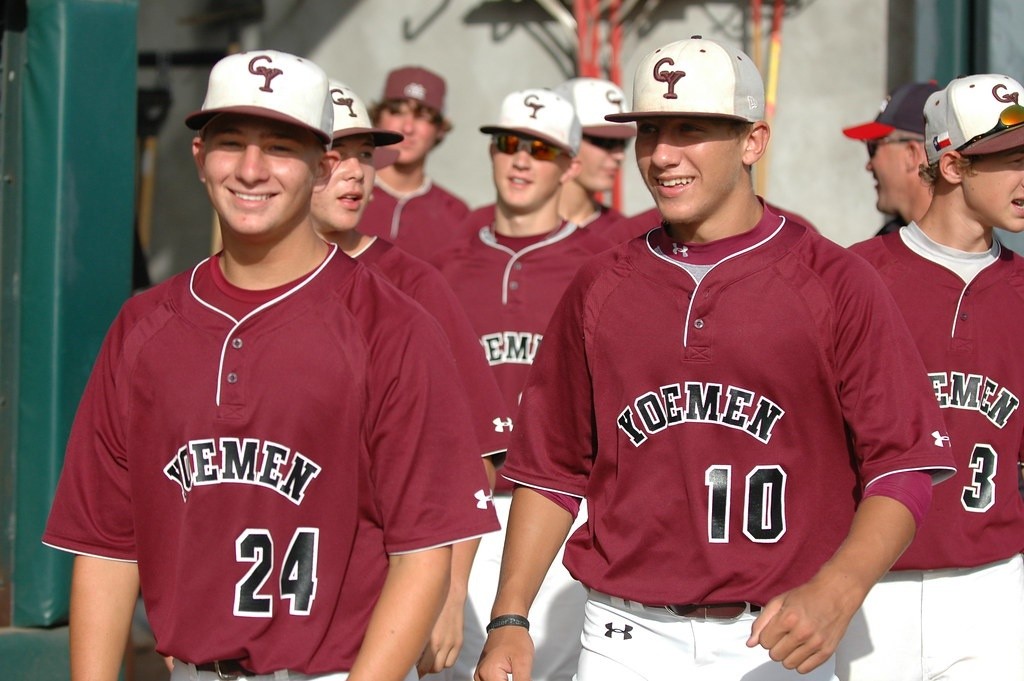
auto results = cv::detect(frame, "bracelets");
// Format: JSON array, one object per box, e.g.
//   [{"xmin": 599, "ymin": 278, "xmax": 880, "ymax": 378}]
[{"xmin": 485, "ymin": 614, "xmax": 530, "ymax": 634}]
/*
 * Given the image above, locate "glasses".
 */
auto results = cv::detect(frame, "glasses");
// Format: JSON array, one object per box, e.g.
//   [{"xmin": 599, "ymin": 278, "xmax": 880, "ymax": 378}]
[
  {"xmin": 867, "ymin": 138, "xmax": 923, "ymax": 157},
  {"xmin": 584, "ymin": 134, "xmax": 626, "ymax": 151},
  {"xmin": 492, "ymin": 132, "xmax": 567, "ymax": 161}
]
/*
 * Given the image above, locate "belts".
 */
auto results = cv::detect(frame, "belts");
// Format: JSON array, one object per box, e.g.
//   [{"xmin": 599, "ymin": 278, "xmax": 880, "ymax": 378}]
[
  {"xmin": 665, "ymin": 601, "xmax": 761, "ymax": 618},
  {"xmin": 195, "ymin": 657, "xmax": 257, "ymax": 681}
]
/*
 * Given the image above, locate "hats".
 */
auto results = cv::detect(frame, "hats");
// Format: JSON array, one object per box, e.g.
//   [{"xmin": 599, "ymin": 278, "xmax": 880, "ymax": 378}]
[
  {"xmin": 843, "ymin": 80, "xmax": 942, "ymax": 139},
  {"xmin": 384, "ymin": 67, "xmax": 444, "ymax": 112},
  {"xmin": 923, "ymin": 73, "xmax": 1024, "ymax": 165},
  {"xmin": 480, "ymin": 88, "xmax": 583, "ymax": 159},
  {"xmin": 329, "ymin": 84, "xmax": 404, "ymax": 147},
  {"xmin": 185, "ymin": 50, "xmax": 335, "ymax": 145},
  {"xmin": 605, "ymin": 35, "xmax": 765, "ymax": 123},
  {"xmin": 553, "ymin": 77, "xmax": 637, "ymax": 140}
]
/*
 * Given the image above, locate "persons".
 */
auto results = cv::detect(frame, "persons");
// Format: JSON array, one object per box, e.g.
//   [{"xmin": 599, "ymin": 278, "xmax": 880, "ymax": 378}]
[
  {"xmin": 838, "ymin": 72, "xmax": 1024, "ymax": 681},
  {"xmin": 38, "ymin": 46, "xmax": 503, "ymax": 681},
  {"xmin": 355, "ymin": 64, "xmax": 949, "ymax": 271},
  {"xmin": 432, "ymin": 88, "xmax": 614, "ymax": 681},
  {"xmin": 471, "ymin": 33, "xmax": 959, "ymax": 681},
  {"xmin": 160, "ymin": 78, "xmax": 510, "ymax": 679}
]
